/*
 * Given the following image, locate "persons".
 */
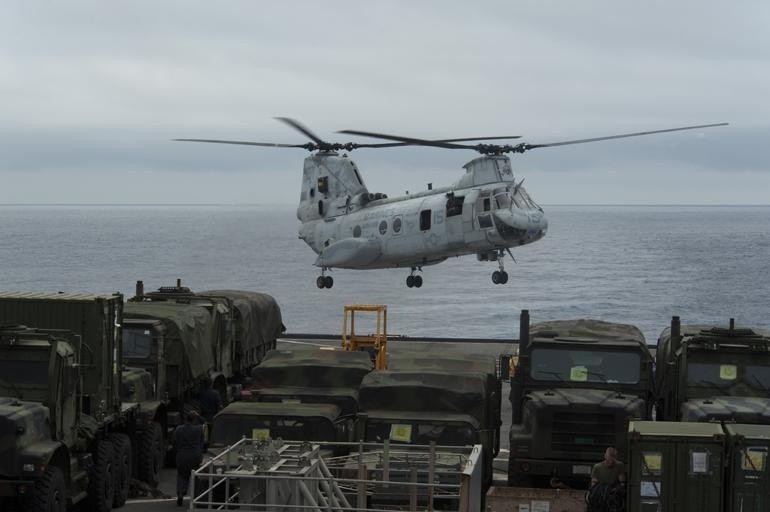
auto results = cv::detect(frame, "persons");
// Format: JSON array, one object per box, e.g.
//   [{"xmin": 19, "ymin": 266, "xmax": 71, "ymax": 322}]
[
  {"xmin": 171, "ymin": 409, "xmax": 204, "ymax": 506},
  {"xmin": 445, "ymin": 190, "xmax": 462, "ymax": 216},
  {"xmin": 585, "ymin": 446, "xmax": 626, "ymax": 512},
  {"xmin": 197, "ymin": 376, "xmax": 224, "ymax": 441}
]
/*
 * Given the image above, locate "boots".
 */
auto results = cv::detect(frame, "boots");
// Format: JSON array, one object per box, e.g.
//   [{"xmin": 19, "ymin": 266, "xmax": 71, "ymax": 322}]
[{"xmin": 176, "ymin": 493, "xmax": 183, "ymax": 506}]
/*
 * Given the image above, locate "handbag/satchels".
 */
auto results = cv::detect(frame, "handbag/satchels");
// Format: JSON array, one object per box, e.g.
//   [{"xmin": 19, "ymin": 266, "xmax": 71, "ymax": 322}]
[{"xmin": 162, "ymin": 446, "xmax": 177, "ymax": 469}]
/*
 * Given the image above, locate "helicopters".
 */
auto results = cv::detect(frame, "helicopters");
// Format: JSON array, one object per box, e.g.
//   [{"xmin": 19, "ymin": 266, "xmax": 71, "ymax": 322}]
[{"xmin": 171, "ymin": 114, "xmax": 728, "ymax": 289}]
